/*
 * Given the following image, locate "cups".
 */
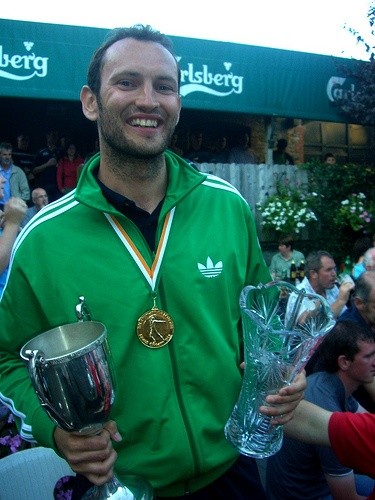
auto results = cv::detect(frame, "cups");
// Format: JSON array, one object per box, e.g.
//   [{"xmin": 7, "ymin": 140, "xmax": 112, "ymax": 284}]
[{"xmin": 224, "ymin": 283, "xmax": 335, "ymax": 456}]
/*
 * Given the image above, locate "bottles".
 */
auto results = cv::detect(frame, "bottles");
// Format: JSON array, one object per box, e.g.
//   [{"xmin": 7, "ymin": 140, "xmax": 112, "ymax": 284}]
[
  {"xmin": 345, "ymin": 255, "xmax": 351, "ymax": 273},
  {"xmin": 300, "ymin": 258, "xmax": 305, "ymax": 277},
  {"xmin": 290, "ymin": 259, "xmax": 296, "ymax": 278}
]
